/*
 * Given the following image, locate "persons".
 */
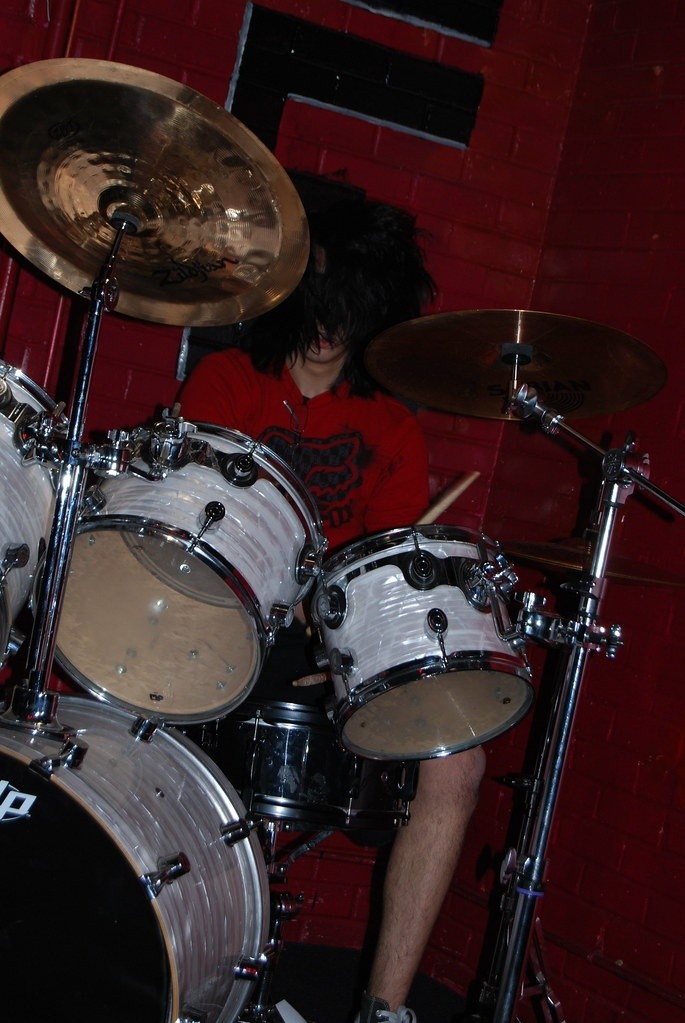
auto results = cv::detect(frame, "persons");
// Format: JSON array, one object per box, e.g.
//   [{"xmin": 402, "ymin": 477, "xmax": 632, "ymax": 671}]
[{"xmin": 177, "ymin": 170, "xmax": 485, "ymax": 1023}]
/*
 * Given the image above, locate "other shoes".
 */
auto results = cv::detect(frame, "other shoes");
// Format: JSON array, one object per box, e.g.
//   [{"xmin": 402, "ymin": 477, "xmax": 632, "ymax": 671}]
[{"xmin": 356, "ymin": 989, "xmax": 415, "ymax": 1023}]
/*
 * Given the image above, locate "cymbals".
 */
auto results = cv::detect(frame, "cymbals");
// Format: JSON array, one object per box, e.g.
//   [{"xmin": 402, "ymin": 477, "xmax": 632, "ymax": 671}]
[
  {"xmin": 367, "ymin": 307, "xmax": 667, "ymax": 422},
  {"xmin": 497, "ymin": 535, "xmax": 678, "ymax": 590},
  {"xmin": 0, "ymin": 57, "xmax": 312, "ymax": 328}
]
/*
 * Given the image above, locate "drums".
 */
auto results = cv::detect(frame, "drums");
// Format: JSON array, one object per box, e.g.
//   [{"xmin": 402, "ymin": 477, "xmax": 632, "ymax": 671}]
[
  {"xmin": 0, "ymin": 695, "xmax": 276, "ymax": 1023},
  {"xmin": 192, "ymin": 697, "xmax": 415, "ymax": 834},
  {"xmin": 51, "ymin": 417, "xmax": 329, "ymax": 729},
  {"xmin": 0, "ymin": 357, "xmax": 68, "ymax": 672},
  {"xmin": 304, "ymin": 524, "xmax": 535, "ymax": 763}
]
